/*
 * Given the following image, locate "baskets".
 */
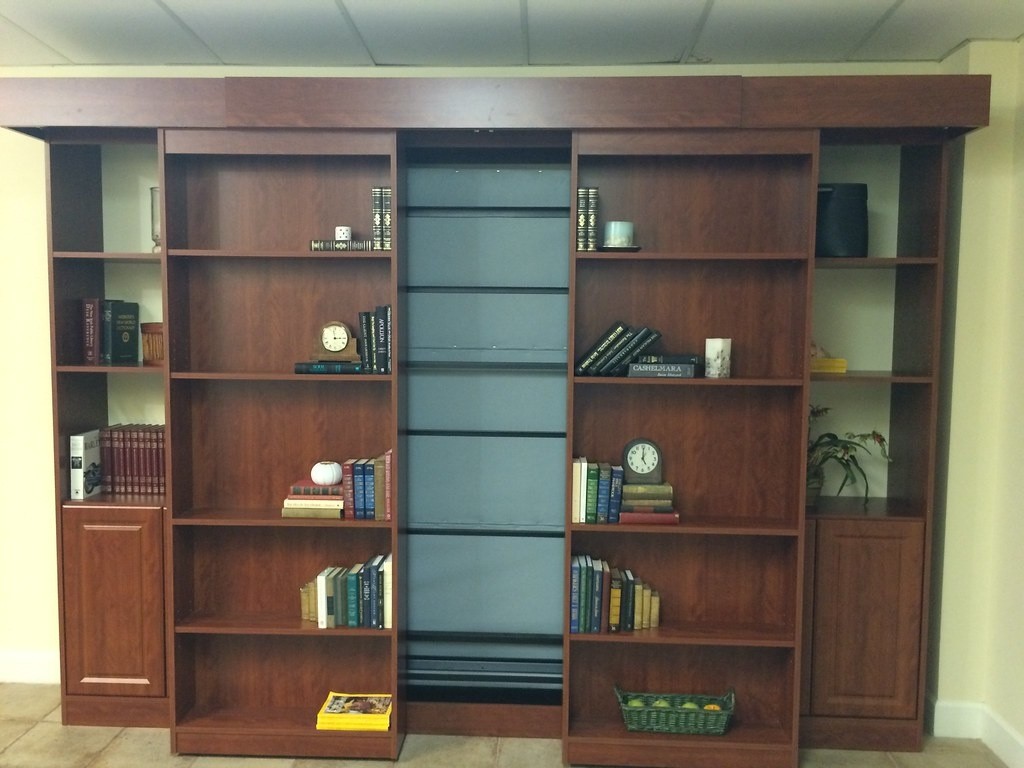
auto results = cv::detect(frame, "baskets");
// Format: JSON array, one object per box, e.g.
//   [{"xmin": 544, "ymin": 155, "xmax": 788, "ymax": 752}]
[{"xmin": 613, "ymin": 680, "xmax": 737, "ymax": 736}]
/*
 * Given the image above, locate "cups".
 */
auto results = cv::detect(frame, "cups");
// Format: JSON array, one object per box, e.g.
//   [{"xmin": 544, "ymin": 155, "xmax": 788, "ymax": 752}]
[
  {"xmin": 705, "ymin": 338, "xmax": 731, "ymax": 378},
  {"xmin": 602, "ymin": 220, "xmax": 633, "ymax": 247}
]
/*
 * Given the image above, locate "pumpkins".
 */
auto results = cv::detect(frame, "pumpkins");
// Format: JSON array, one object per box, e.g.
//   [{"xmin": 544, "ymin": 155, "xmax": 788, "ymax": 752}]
[{"xmin": 311, "ymin": 461, "xmax": 343, "ymax": 485}]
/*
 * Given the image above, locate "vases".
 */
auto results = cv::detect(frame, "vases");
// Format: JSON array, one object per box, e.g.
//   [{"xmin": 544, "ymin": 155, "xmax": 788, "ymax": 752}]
[{"xmin": 805, "ymin": 479, "xmax": 821, "ymax": 504}]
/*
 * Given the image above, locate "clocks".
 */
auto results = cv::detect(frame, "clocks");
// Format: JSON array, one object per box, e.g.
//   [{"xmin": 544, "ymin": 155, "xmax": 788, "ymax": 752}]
[
  {"xmin": 620, "ymin": 439, "xmax": 662, "ymax": 485},
  {"xmin": 308, "ymin": 321, "xmax": 361, "ymax": 365}
]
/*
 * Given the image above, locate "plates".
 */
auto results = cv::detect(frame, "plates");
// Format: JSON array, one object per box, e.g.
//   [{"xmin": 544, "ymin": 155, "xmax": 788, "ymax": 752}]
[{"xmin": 597, "ymin": 246, "xmax": 643, "ymax": 252}]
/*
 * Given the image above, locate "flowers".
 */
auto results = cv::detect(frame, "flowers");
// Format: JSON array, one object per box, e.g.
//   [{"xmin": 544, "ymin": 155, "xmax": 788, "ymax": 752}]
[{"xmin": 804, "ymin": 405, "xmax": 891, "ymax": 505}]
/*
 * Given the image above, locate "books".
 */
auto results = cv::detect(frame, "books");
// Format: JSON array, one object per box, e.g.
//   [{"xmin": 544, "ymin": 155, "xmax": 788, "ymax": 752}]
[
  {"xmin": 282, "ymin": 447, "xmax": 392, "ymax": 521},
  {"xmin": 70, "ymin": 423, "xmax": 166, "ymax": 500},
  {"xmin": 311, "ymin": 239, "xmax": 372, "ymax": 251},
  {"xmin": 808, "ymin": 337, "xmax": 847, "ymax": 373},
  {"xmin": 371, "ymin": 186, "xmax": 392, "ymax": 250},
  {"xmin": 294, "ymin": 363, "xmax": 362, "ymax": 374},
  {"xmin": 570, "ymin": 553, "xmax": 660, "ymax": 635},
  {"xmin": 316, "ymin": 691, "xmax": 391, "ymax": 731},
  {"xmin": 572, "ymin": 457, "xmax": 680, "ymax": 525},
  {"xmin": 299, "ymin": 553, "xmax": 392, "ymax": 629},
  {"xmin": 359, "ymin": 304, "xmax": 392, "ymax": 375},
  {"xmin": 818, "ymin": 182, "xmax": 869, "ymax": 257},
  {"xmin": 578, "ymin": 187, "xmax": 597, "ymax": 251},
  {"xmin": 576, "ymin": 322, "xmax": 705, "ymax": 377},
  {"xmin": 78, "ymin": 295, "xmax": 140, "ymax": 363}
]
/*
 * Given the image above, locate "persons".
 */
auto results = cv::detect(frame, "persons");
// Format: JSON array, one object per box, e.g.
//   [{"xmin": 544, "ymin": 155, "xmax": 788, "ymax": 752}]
[{"xmin": 344, "ymin": 699, "xmax": 380, "ymax": 713}]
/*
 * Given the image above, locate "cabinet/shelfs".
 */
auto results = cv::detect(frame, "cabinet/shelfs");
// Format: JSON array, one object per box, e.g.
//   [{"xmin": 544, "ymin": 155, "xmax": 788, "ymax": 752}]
[
  {"xmin": 799, "ymin": 123, "xmax": 956, "ymax": 752},
  {"xmin": 158, "ymin": 131, "xmax": 406, "ymax": 763},
  {"xmin": 46, "ymin": 134, "xmax": 169, "ymax": 727},
  {"xmin": 559, "ymin": 125, "xmax": 818, "ymax": 767}
]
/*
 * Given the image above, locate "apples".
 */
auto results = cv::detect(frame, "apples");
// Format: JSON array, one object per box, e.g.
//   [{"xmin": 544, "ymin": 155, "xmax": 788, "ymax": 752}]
[
  {"xmin": 652, "ymin": 700, "xmax": 670, "ymax": 707},
  {"xmin": 629, "ymin": 700, "xmax": 644, "ymax": 706},
  {"xmin": 682, "ymin": 702, "xmax": 699, "ymax": 708},
  {"xmin": 704, "ymin": 704, "xmax": 720, "ymax": 710}
]
